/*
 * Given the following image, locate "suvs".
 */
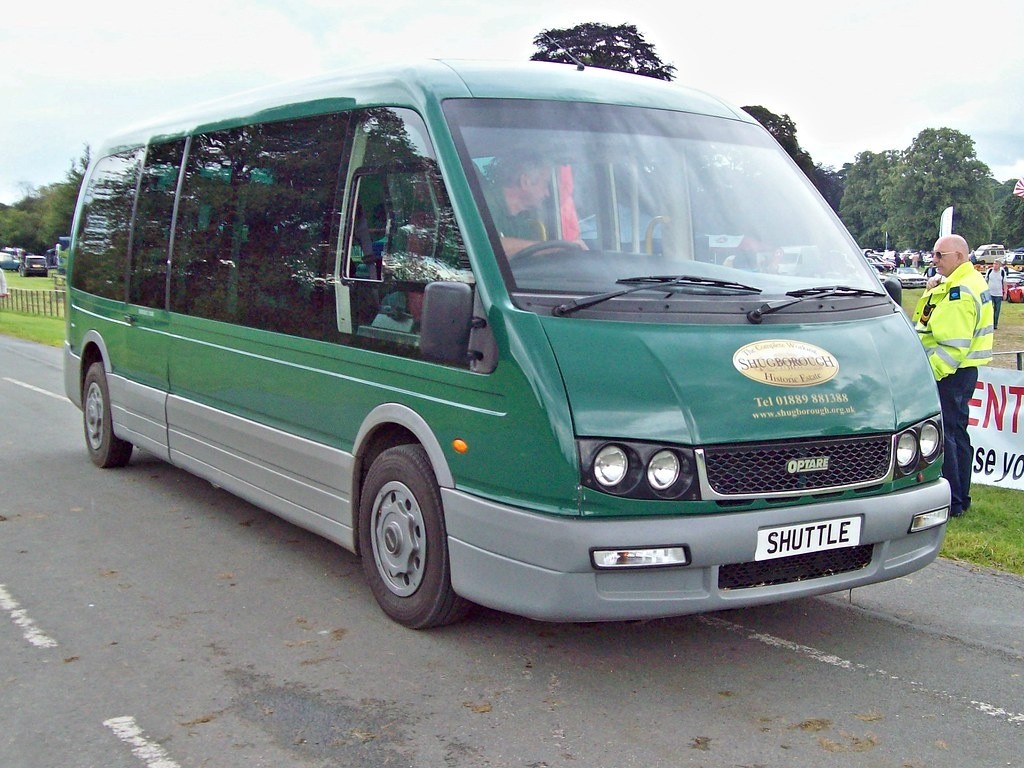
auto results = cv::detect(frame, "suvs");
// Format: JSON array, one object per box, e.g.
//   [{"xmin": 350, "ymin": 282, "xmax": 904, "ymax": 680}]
[{"xmin": 18, "ymin": 256, "xmax": 48, "ymax": 277}]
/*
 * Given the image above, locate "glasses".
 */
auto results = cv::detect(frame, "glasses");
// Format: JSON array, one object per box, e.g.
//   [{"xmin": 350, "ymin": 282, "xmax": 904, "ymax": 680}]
[{"xmin": 932, "ymin": 251, "xmax": 958, "ymax": 259}]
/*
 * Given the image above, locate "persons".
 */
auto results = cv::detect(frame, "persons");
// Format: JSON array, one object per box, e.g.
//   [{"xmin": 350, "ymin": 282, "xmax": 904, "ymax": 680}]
[
  {"xmin": 483, "ymin": 145, "xmax": 590, "ymax": 262},
  {"xmin": 969, "ymin": 250, "xmax": 977, "ymax": 265},
  {"xmin": 911, "ymin": 234, "xmax": 994, "ymax": 518},
  {"xmin": 867, "ymin": 247, "xmax": 934, "ymax": 275},
  {"xmin": 922, "ymin": 261, "xmax": 937, "ymax": 281},
  {"xmin": 985, "ymin": 259, "xmax": 1007, "ymax": 330},
  {"xmin": 0, "ymin": 268, "xmax": 11, "ymax": 300},
  {"xmin": 875, "ymin": 265, "xmax": 902, "ymax": 307},
  {"xmin": 722, "ymin": 234, "xmax": 788, "ymax": 274}
]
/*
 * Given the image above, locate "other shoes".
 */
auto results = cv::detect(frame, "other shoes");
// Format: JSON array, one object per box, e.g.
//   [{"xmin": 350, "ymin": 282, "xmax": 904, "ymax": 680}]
[
  {"xmin": 950, "ymin": 513, "xmax": 962, "ymax": 517},
  {"xmin": 994, "ymin": 327, "xmax": 997, "ymax": 330}
]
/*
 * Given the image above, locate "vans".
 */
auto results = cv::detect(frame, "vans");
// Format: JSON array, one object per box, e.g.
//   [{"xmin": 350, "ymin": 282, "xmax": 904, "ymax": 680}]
[
  {"xmin": 1006, "ymin": 254, "xmax": 1024, "ymax": 265},
  {"xmin": 968, "ymin": 243, "xmax": 1006, "ymax": 264},
  {"xmin": 66, "ymin": 58, "xmax": 952, "ymax": 629}
]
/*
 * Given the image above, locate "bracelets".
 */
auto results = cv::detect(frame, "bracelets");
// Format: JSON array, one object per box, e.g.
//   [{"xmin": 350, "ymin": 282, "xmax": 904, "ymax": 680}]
[{"xmin": 1003, "ymin": 291, "xmax": 1007, "ymax": 294}]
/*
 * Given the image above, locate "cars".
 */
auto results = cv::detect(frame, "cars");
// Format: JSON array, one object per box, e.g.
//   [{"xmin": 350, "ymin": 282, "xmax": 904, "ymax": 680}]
[
  {"xmin": 0, "ymin": 247, "xmax": 34, "ymax": 273},
  {"xmin": 861, "ymin": 248, "xmax": 936, "ymax": 289},
  {"xmin": 979, "ymin": 266, "xmax": 1024, "ymax": 303}
]
